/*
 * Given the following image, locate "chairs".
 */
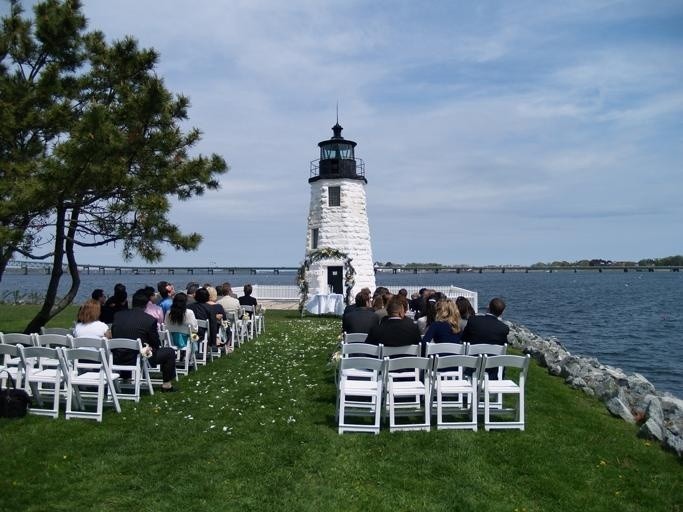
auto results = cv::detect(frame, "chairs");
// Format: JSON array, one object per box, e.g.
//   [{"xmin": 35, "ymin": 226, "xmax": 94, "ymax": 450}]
[
  {"xmin": 336, "ymin": 308, "xmax": 531, "ymax": 435},
  {"xmin": 0, "ymin": 302, "xmax": 266, "ymax": 422},
  {"xmin": 304, "ymin": 288, "xmax": 320, "ymax": 303}
]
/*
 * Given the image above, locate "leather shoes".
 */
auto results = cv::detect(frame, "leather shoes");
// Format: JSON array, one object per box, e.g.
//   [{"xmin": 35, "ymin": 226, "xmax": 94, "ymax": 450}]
[{"xmin": 162, "ymin": 386, "xmax": 179, "ymax": 392}]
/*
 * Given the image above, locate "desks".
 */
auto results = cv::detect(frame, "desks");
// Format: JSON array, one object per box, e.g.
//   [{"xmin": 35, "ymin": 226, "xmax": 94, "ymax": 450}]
[{"xmin": 304, "ymin": 293, "xmax": 347, "ymax": 316}]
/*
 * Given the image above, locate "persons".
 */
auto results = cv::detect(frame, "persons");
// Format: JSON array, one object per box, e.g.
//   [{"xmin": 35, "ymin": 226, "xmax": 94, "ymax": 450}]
[
  {"xmin": 75, "ymin": 281, "xmax": 258, "ymax": 393},
  {"xmin": 342, "ymin": 287, "xmax": 511, "ymax": 379}
]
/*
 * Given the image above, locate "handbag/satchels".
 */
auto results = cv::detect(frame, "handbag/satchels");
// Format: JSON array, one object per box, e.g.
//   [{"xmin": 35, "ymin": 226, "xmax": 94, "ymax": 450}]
[{"xmin": 0, "ymin": 370, "xmax": 27, "ymax": 418}]
